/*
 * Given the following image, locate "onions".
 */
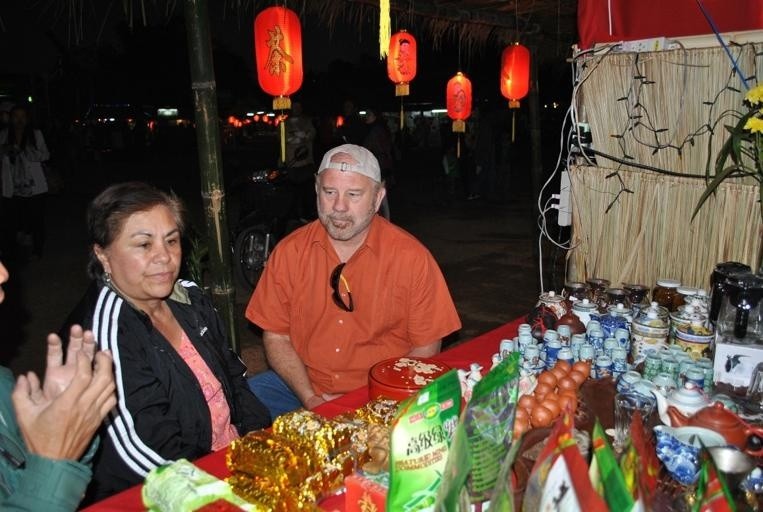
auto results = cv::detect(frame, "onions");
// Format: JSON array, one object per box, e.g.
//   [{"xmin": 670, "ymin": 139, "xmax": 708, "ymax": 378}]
[{"xmin": 513, "ymin": 362, "xmax": 590, "ymax": 439}]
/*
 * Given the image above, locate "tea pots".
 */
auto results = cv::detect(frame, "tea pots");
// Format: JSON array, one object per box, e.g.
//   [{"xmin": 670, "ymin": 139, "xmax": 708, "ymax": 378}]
[
  {"xmin": 650, "ymin": 382, "xmax": 763, "ymax": 488},
  {"xmin": 715, "ymin": 271, "xmax": 762, "ymax": 346},
  {"xmin": 706, "ymin": 261, "xmax": 753, "ymax": 326}
]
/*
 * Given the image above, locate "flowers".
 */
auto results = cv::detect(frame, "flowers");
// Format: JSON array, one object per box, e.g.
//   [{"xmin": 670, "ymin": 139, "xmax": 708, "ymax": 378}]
[{"xmin": 690, "ymin": 87, "xmax": 763, "ymax": 219}]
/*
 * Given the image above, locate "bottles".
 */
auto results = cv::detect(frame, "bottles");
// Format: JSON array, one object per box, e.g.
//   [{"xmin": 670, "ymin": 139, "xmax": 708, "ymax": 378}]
[{"xmin": 367, "ymin": 355, "xmax": 452, "ymax": 476}]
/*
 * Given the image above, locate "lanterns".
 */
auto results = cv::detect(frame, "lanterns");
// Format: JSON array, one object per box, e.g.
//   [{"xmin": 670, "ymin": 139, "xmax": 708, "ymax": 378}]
[
  {"xmin": 499, "ymin": 42, "xmax": 532, "ymax": 142},
  {"xmin": 253, "ymin": 6, "xmax": 304, "ymax": 164},
  {"xmin": 446, "ymin": 72, "xmax": 473, "ymax": 158},
  {"xmin": 386, "ymin": 28, "xmax": 418, "ymax": 131}
]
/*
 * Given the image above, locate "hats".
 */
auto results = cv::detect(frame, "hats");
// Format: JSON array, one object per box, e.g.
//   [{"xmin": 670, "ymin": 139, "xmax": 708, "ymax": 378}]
[{"xmin": 317, "ymin": 144, "xmax": 381, "ymax": 183}]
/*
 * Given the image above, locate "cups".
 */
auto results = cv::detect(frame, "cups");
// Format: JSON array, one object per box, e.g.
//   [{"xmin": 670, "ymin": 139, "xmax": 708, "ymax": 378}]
[
  {"xmin": 612, "ymin": 390, "xmax": 655, "ymax": 448},
  {"xmin": 492, "ymin": 290, "xmax": 713, "ymax": 421}
]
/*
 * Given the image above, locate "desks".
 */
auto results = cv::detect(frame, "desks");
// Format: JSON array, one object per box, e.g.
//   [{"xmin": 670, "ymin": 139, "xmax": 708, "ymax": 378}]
[{"xmin": 65, "ymin": 307, "xmax": 763, "ymax": 512}]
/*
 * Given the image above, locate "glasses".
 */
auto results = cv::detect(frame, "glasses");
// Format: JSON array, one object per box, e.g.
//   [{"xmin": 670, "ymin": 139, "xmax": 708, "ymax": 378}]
[{"xmin": 330, "ymin": 263, "xmax": 353, "ymax": 312}]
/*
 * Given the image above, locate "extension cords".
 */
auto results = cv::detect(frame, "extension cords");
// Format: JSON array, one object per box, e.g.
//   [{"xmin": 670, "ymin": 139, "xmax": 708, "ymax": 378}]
[
  {"xmin": 558, "ymin": 171, "xmax": 573, "ymax": 227},
  {"xmin": 571, "ymin": 122, "xmax": 589, "ymax": 166}
]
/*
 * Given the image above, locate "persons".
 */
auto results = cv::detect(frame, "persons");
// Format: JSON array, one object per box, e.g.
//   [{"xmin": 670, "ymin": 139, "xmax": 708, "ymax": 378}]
[
  {"xmin": 58, "ymin": 178, "xmax": 272, "ymax": 512},
  {"xmin": 245, "ymin": 143, "xmax": 462, "ymax": 424},
  {"xmin": 0, "ymin": 262, "xmax": 118, "ymax": 512},
  {"xmin": 0, "ymin": 105, "xmax": 50, "ymax": 263},
  {"xmin": 0, "ymin": 100, "xmax": 16, "ymax": 123},
  {"xmin": 285, "ymin": 98, "xmax": 393, "ymax": 223}
]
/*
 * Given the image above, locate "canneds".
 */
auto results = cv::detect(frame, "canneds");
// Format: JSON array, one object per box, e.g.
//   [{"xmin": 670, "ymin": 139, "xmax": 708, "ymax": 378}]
[{"xmin": 652, "ymin": 280, "xmax": 698, "ymax": 314}]
